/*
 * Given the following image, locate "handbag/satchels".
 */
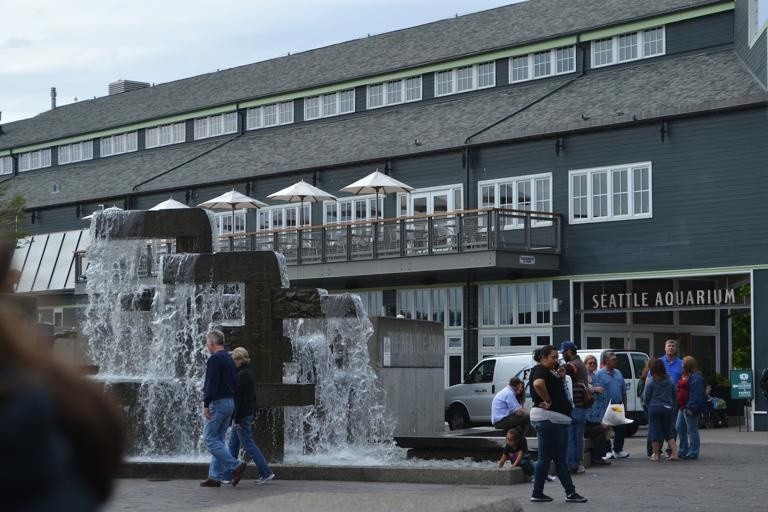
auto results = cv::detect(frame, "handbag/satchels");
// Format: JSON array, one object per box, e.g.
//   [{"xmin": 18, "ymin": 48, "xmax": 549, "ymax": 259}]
[{"xmin": 582, "ymin": 386, "xmax": 595, "ymax": 409}]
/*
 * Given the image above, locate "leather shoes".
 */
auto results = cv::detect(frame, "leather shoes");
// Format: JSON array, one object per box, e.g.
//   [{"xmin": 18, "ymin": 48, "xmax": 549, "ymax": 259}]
[
  {"xmin": 230, "ymin": 461, "xmax": 247, "ymax": 487},
  {"xmin": 646, "ymin": 448, "xmax": 697, "ymax": 461},
  {"xmin": 591, "ymin": 458, "xmax": 611, "ymax": 465},
  {"xmin": 200, "ymin": 478, "xmax": 221, "ymax": 487}
]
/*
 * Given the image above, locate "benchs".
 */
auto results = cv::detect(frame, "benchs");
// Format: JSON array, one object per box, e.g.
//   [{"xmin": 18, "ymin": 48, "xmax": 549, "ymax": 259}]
[{"xmin": 392, "ymin": 425, "xmax": 592, "ymax": 460}]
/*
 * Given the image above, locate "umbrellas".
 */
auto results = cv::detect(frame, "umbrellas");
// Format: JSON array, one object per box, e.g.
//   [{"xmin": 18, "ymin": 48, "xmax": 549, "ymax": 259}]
[
  {"xmin": 268, "ymin": 176, "xmax": 338, "ymax": 223},
  {"xmin": 197, "ymin": 188, "xmax": 269, "ymax": 232},
  {"xmin": 150, "ymin": 196, "xmax": 190, "ymax": 208},
  {"xmin": 341, "ymin": 168, "xmax": 415, "ymax": 217}
]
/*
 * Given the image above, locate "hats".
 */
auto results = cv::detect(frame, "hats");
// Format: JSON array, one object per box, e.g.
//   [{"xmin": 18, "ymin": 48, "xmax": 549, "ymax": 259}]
[{"xmin": 558, "ymin": 340, "xmax": 577, "ymax": 354}]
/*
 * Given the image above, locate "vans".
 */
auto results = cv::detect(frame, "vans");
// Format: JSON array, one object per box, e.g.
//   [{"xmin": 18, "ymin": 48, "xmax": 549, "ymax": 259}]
[{"xmin": 444, "ymin": 347, "xmax": 654, "ymax": 437}]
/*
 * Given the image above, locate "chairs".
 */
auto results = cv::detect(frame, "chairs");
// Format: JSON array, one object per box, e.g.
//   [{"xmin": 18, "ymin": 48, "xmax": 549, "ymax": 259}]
[{"xmin": 214, "ymin": 209, "xmax": 508, "ymax": 264}]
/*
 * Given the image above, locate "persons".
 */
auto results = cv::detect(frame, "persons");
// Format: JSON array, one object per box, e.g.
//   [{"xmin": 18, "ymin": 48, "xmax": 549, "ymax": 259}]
[
  {"xmin": 230, "ymin": 346, "xmax": 275, "ymax": 484},
  {"xmin": 485, "ymin": 338, "xmax": 726, "ymax": 498},
  {"xmin": 1, "ymin": 225, "xmax": 123, "ymax": 512},
  {"xmin": 201, "ymin": 329, "xmax": 246, "ymax": 484}
]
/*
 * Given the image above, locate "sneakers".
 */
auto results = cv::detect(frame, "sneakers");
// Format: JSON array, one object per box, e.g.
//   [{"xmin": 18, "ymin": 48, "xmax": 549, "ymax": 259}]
[
  {"xmin": 547, "ymin": 473, "xmax": 557, "ymax": 481},
  {"xmin": 602, "ymin": 451, "xmax": 615, "ymax": 460},
  {"xmin": 253, "ymin": 472, "xmax": 276, "ymax": 485},
  {"xmin": 220, "ymin": 479, "xmax": 233, "ymax": 484},
  {"xmin": 530, "ymin": 494, "xmax": 554, "ymax": 502},
  {"xmin": 612, "ymin": 449, "xmax": 630, "ymax": 458},
  {"xmin": 529, "ymin": 474, "xmax": 535, "ymax": 482},
  {"xmin": 564, "ymin": 493, "xmax": 587, "ymax": 503}
]
